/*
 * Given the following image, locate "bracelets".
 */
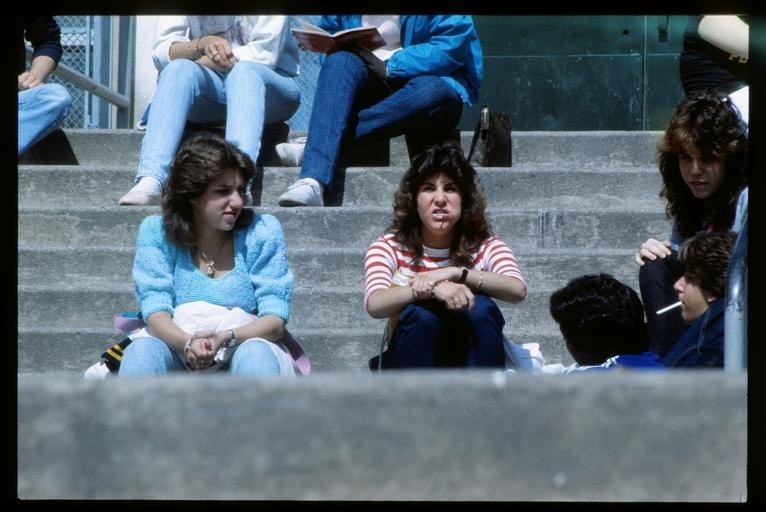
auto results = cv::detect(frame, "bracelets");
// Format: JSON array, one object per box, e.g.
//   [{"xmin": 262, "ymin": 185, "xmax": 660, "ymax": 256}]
[
  {"xmin": 194, "ymin": 35, "xmax": 204, "ymax": 58},
  {"xmin": 181, "ymin": 336, "xmax": 195, "ymax": 357},
  {"xmin": 475, "ymin": 269, "xmax": 484, "ymax": 290}
]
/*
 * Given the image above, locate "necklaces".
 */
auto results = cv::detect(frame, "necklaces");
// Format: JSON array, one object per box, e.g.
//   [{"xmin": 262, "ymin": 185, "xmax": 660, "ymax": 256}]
[{"xmin": 186, "ymin": 229, "xmax": 233, "ymax": 278}]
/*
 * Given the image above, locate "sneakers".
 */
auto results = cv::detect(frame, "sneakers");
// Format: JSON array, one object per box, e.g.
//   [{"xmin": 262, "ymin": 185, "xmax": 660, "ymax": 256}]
[
  {"xmin": 278, "ymin": 178, "xmax": 326, "ymax": 207},
  {"xmin": 275, "ymin": 137, "xmax": 307, "ymax": 167},
  {"xmin": 119, "ymin": 180, "xmax": 163, "ymax": 206},
  {"xmin": 243, "ymin": 190, "xmax": 253, "ymax": 206}
]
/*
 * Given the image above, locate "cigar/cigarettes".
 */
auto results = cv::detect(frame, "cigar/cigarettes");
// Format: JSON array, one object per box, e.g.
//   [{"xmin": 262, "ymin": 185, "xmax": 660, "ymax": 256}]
[{"xmin": 655, "ymin": 300, "xmax": 684, "ymax": 315}]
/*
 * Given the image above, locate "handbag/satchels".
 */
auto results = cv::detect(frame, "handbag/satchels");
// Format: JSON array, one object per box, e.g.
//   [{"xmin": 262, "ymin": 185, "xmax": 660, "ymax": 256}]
[{"xmin": 465, "ymin": 103, "xmax": 512, "ymax": 168}]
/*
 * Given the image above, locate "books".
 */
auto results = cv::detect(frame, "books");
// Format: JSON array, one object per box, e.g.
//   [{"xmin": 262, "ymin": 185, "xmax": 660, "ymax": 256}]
[{"xmin": 284, "ymin": 16, "xmax": 388, "ymax": 56}]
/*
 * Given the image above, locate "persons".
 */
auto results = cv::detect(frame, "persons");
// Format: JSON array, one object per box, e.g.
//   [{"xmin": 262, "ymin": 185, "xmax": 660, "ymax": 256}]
[
  {"xmin": 16, "ymin": 15, "xmax": 74, "ymax": 164},
  {"xmin": 677, "ymin": 15, "xmax": 750, "ymax": 134},
  {"xmin": 277, "ymin": 12, "xmax": 485, "ymax": 209},
  {"xmin": 113, "ymin": 131, "xmax": 310, "ymax": 378},
  {"xmin": 361, "ymin": 141, "xmax": 531, "ymax": 371},
  {"xmin": 652, "ymin": 229, "xmax": 739, "ymax": 372},
  {"xmin": 114, "ymin": 15, "xmax": 303, "ymax": 209},
  {"xmin": 633, "ymin": 88, "xmax": 751, "ymax": 360},
  {"xmin": 543, "ymin": 271, "xmax": 666, "ymax": 369}
]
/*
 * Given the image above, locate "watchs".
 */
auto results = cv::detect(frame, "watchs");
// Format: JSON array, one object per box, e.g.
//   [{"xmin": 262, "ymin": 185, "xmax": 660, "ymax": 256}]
[
  {"xmin": 221, "ymin": 328, "xmax": 238, "ymax": 349},
  {"xmin": 456, "ymin": 265, "xmax": 469, "ymax": 283}
]
